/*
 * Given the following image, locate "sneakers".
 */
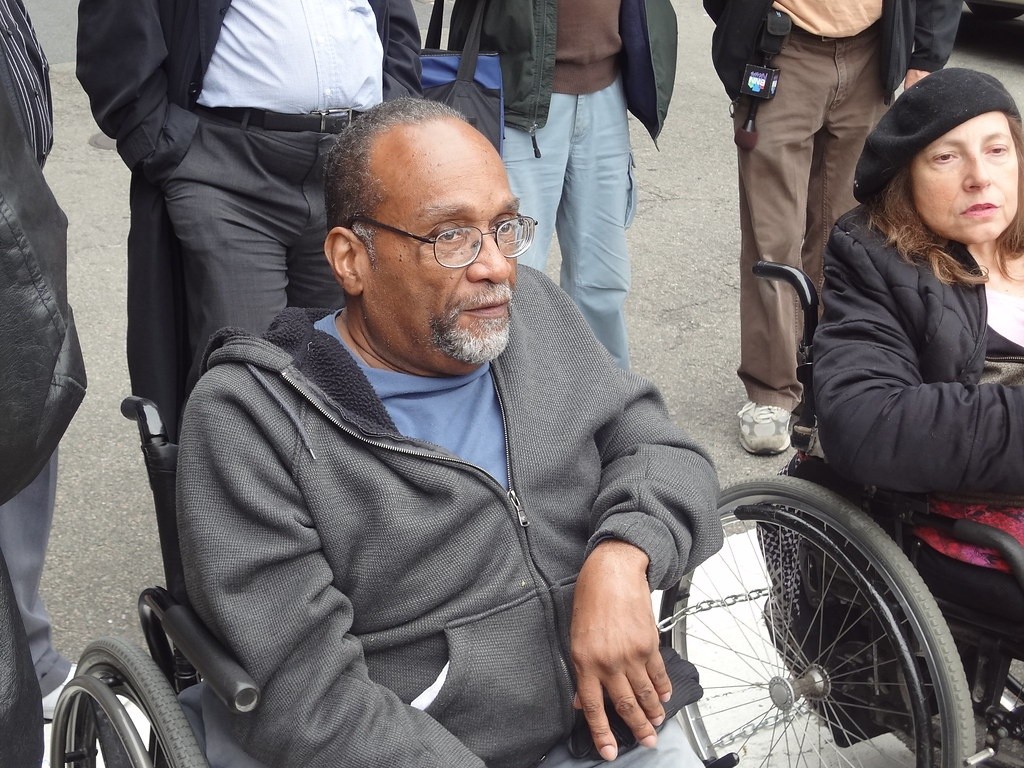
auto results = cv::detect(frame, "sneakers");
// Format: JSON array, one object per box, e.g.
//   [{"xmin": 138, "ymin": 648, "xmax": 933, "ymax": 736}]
[{"xmin": 737, "ymin": 401, "xmax": 791, "ymax": 455}]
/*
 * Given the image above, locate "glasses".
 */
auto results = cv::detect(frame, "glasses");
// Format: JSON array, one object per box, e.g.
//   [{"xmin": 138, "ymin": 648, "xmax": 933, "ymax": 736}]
[{"xmin": 346, "ymin": 216, "xmax": 538, "ymax": 269}]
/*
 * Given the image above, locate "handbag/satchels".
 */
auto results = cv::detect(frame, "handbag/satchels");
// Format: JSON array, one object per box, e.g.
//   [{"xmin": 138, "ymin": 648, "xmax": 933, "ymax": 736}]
[{"xmin": 420, "ymin": 0, "xmax": 505, "ymax": 159}]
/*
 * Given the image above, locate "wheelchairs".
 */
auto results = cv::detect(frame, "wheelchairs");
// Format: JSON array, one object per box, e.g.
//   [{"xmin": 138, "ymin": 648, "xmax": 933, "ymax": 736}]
[
  {"xmin": 47, "ymin": 397, "xmax": 978, "ymax": 768},
  {"xmin": 747, "ymin": 258, "xmax": 1023, "ymax": 768}
]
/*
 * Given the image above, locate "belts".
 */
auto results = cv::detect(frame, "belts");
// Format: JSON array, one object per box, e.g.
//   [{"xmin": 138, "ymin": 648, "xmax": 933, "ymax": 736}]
[
  {"xmin": 197, "ymin": 103, "xmax": 363, "ymax": 135},
  {"xmin": 790, "ymin": 23, "xmax": 837, "ymax": 42}
]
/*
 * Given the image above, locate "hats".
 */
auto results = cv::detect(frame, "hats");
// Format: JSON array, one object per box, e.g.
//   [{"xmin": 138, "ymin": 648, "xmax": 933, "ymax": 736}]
[{"xmin": 853, "ymin": 68, "xmax": 1021, "ymax": 204}]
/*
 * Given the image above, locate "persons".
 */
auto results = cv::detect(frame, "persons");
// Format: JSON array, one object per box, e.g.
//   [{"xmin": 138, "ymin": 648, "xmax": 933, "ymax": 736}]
[
  {"xmin": 812, "ymin": 66, "xmax": 1024, "ymax": 627},
  {"xmin": 74, "ymin": 0, "xmax": 424, "ymax": 445},
  {"xmin": 1, "ymin": 1, "xmax": 146, "ymax": 728},
  {"xmin": 175, "ymin": 96, "xmax": 725, "ymax": 767},
  {"xmin": 447, "ymin": 0, "xmax": 679, "ymax": 374},
  {"xmin": 703, "ymin": 0, "xmax": 965, "ymax": 458}
]
